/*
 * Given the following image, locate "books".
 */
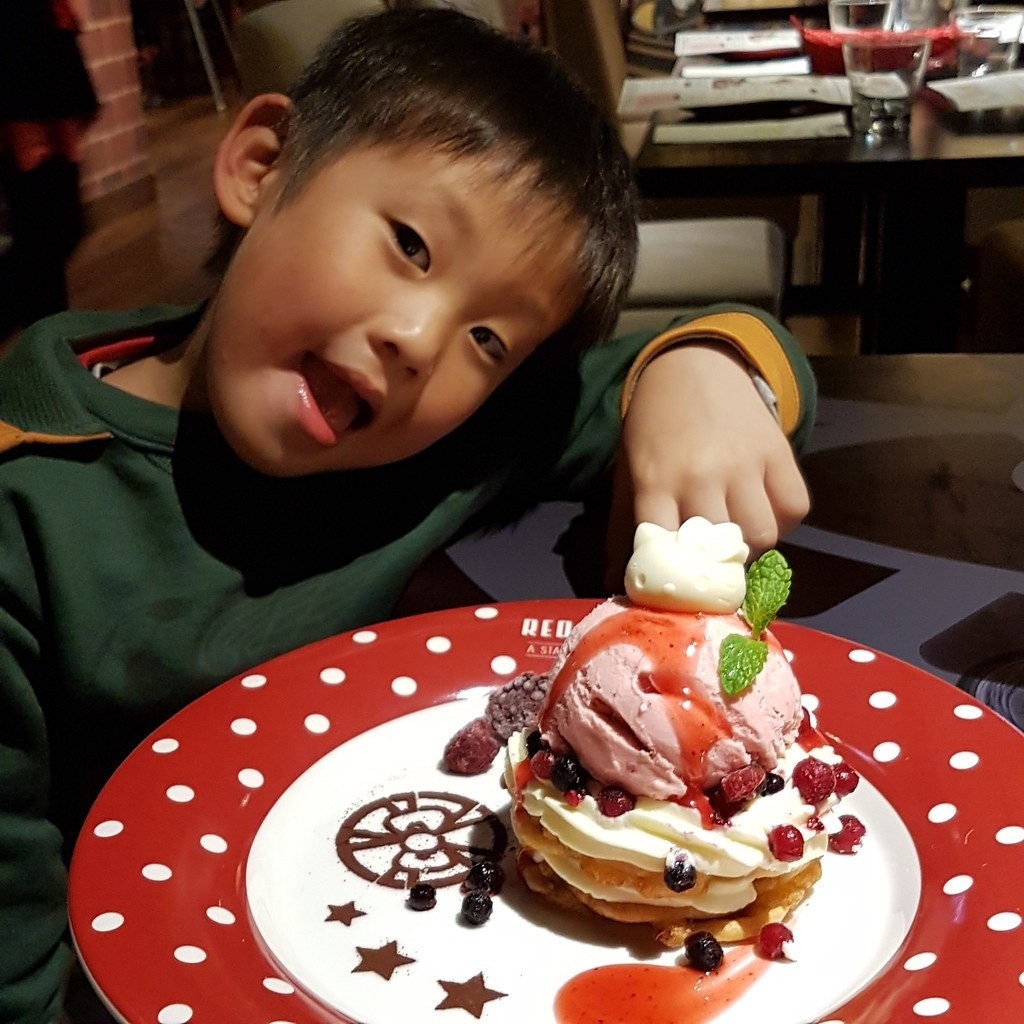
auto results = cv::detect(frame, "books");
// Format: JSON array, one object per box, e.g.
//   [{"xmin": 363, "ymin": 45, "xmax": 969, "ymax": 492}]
[{"xmin": 649, "ymin": 97, "xmax": 850, "ymax": 144}]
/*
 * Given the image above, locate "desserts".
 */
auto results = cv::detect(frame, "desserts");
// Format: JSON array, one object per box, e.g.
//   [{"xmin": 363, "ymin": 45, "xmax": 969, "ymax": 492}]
[{"xmin": 494, "ymin": 516, "xmax": 860, "ymax": 941}]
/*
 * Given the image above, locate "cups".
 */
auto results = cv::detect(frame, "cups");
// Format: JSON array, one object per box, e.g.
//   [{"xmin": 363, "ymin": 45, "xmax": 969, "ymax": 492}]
[
  {"xmin": 828, "ymin": 0, "xmax": 969, "ymax": 32},
  {"xmin": 841, "ymin": 36, "xmax": 930, "ymax": 136},
  {"xmin": 953, "ymin": 6, "xmax": 1024, "ymax": 77}
]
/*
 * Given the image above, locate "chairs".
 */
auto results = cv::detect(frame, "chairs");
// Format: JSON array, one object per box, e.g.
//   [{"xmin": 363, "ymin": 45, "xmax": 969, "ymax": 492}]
[
  {"xmin": 540, "ymin": 0, "xmax": 802, "ymax": 282},
  {"xmin": 230, "ymin": 1, "xmax": 785, "ymax": 340}
]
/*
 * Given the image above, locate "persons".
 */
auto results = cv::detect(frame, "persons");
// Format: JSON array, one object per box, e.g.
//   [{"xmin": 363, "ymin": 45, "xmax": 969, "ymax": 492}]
[{"xmin": 1, "ymin": 8, "xmax": 822, "ymax": 1024}]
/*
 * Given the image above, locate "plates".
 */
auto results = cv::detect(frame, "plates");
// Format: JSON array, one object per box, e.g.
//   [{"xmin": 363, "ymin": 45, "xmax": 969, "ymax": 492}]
[{"xmin": 63, "ymin": 596, "xmax": 1024, "ymax": 1024}]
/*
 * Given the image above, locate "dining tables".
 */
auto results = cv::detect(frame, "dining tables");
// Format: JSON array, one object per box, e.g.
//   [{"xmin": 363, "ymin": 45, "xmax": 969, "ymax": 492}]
[
  {"xmin": 388, "ymin": 352, "xmax": 1022, "ymax": 731},
  {"xmin": 631, "ymin": 58, "xmax": 1023, "ymax": 353},
  {"xmin": 701, "ymin": 1, "xmax": 885, "ymax": 31}
]
